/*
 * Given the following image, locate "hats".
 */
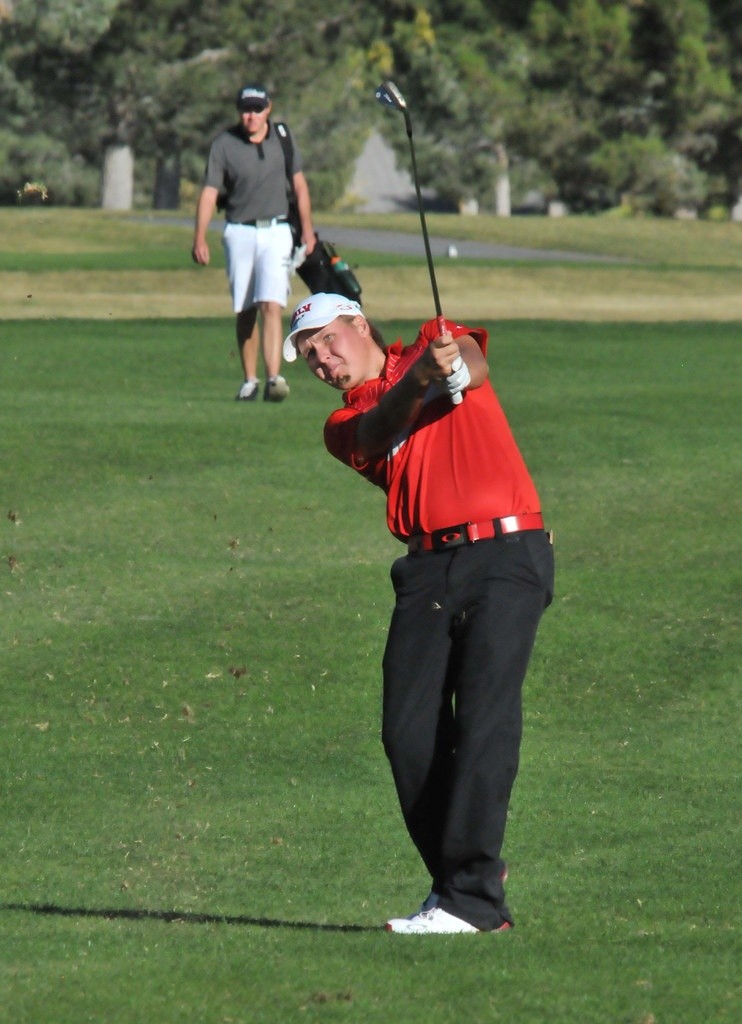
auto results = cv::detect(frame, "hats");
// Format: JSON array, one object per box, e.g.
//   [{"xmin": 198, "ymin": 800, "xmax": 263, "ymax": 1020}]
[
  {"xmin": 239, "ymin": 86, "xmax": 269, "ymax": 106},
  {"xmin": 282, "ymin": 293, "xmax": 365, "ymax": 362}
]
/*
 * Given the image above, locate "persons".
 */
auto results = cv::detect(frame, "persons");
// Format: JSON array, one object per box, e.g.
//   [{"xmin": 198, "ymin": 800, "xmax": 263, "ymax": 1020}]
[
  {"xmin": 281, "ymin": 292, "xmax": 556, "ymax": 935},
  {"xmin": 190, "ymin": 83, "xmax": 316, "ymax": 402}
]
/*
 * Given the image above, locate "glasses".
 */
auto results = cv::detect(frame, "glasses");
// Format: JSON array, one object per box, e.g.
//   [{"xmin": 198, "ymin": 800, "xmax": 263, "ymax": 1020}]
[{"xmin": 243, "ymin": 105, "xmax": 265, "ymax": 113}]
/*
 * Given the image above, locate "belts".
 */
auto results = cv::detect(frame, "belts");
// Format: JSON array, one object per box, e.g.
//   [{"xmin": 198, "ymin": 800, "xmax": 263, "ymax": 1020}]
[
  {"xmin": 229, "ymin": 218, "xmax": 288, "ymax": 228},
  {"xmin": 408, "ymin": 513, "xmax": 544, "ymax": 554}
]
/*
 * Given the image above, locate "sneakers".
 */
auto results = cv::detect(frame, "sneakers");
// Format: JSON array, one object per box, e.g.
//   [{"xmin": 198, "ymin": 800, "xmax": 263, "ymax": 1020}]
[
  {"xmin": 405, "ymin": 858, "xmax": 509, "ymax": 921},
  {"xmin": 386, "ymin": 906, "xmax": 511, "ymax": 936}
]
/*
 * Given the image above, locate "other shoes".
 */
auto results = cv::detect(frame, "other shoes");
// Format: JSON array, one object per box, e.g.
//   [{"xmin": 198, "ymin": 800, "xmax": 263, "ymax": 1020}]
[
  {"xmin": 237, "ymin": 377, "xmax": 260, "ymax": 399},
  {"xmin": 264, "ymin": 374, "xmax": 290, "ymax": 402}
]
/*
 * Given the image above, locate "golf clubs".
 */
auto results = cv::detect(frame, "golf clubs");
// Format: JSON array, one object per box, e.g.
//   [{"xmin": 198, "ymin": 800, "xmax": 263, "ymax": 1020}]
[{"xmin": 375, "ymin": 79, "xmax": 465, "ymax": 406}]
